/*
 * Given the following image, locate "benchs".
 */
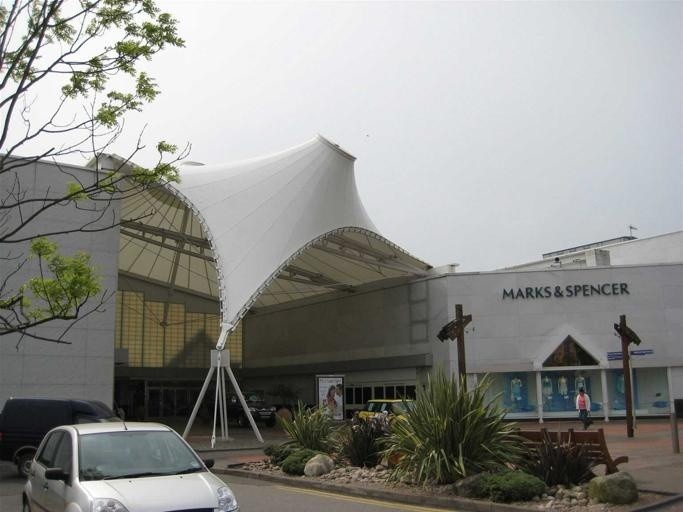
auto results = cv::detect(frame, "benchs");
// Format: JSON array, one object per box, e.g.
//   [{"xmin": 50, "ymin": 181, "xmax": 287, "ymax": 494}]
[{"xmin": 513, "ymin": 428, "xmax": 628, "ymax": 474}]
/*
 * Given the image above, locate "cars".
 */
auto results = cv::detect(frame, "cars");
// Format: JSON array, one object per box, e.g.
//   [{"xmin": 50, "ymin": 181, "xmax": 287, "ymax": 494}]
[{"xmin": 23, "ymin": 421, "xmax": 239, "ymax": 512}]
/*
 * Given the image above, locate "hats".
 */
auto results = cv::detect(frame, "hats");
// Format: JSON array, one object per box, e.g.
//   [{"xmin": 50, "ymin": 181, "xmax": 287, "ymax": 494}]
[{"xmin": 579, "ymin": 387, "xmax": 584, "ymax": 391}]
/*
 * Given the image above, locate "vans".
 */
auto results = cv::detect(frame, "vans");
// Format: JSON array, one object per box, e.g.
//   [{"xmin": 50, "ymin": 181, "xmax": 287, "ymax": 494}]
[
  {"xmin": 356, "ymin": 399, "xmax": 417, "ymax": 436},
  {"xmin": 1, "ymin": 398, "xmax": 125, "ymax": 478}
]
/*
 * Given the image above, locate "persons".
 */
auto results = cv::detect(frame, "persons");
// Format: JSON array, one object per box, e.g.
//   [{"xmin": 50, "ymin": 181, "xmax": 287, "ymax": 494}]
[
  {"xmin": 541, "ymin": 375, "xmax": 553, "ymax": 398},
  {"xmin": 575, "ymin": 389, "xmax": 591, "ymax": 430},
  {"xmin": 323, "ymin": 384, "xmax": 343, "ymax": 419},
  {"xmin": 616, "ymin": 372, "xmax": 625, "ymax": 395},
  {"xmin": 651, "ymin": 392, "xmax": 669, "ymax": 409},
  {"xmin": 557, "ymin": 374, "xmax": 568, "ymax": 396},
  {"xmin": 509, "ymin": 377, "xmax": 522, "ymax": 401},
  {"xmin": 574, "ymin": 374, "xmax": 587, "ymax": 394}
]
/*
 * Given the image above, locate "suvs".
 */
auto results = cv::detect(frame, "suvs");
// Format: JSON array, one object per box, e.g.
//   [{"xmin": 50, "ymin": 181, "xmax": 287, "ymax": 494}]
[{"xmin": 207, "ymin": 391, "xmax": 275, "ymax": 428}]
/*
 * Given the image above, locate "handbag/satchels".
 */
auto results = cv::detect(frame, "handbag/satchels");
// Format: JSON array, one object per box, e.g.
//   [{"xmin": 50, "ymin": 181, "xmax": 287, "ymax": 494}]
[{"xmin": 585, "ymin": 417, "xmax": 593, "ymax": 424}]
